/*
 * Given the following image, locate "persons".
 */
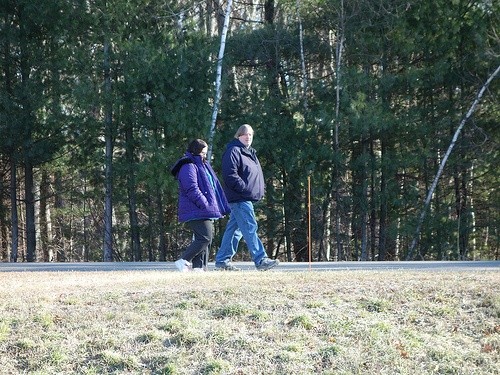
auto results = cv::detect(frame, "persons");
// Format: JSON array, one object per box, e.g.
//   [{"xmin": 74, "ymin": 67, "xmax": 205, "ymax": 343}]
[
  {"xmin": 215, "ymin": 124, "xmax": 280, "ymax": 272},
  {"xmin": 171, "ymin": 138, "xmax": 218, "ymax": 275}
]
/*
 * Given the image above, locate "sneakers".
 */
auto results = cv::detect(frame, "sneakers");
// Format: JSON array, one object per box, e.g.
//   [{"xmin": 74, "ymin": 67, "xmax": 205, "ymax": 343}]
[
  {"xmin": 175, "ymin": 259, "xmax": 194, "ymax": 271},
  {"xmin": 193, "ymin": 267, "xmax": 205, "ymax": 272},
  {"xmin": 215, "ymin": 265, "xmax": 241, "ymax": 271},
  {"xmin": 255, "ymin": 257, "xmax": 280, "ymax": 271}
]
